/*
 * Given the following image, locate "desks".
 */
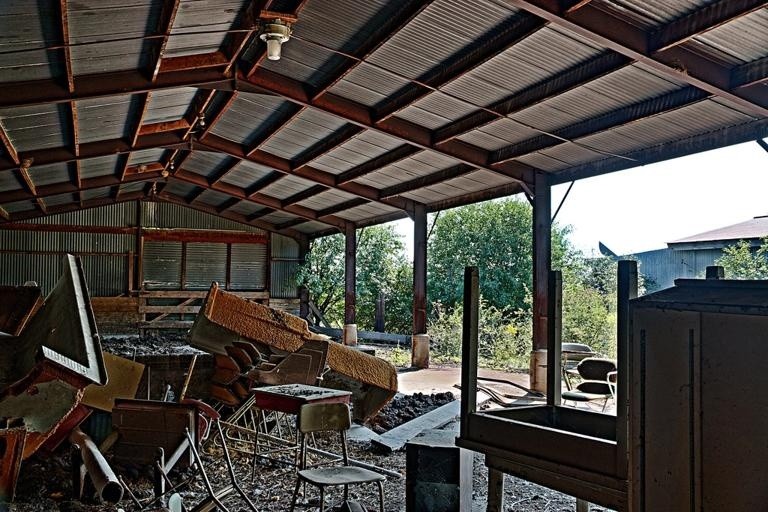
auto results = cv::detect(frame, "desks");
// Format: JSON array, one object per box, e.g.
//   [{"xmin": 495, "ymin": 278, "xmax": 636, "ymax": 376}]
[
  {"xmin": 250, "ymin": 383, "xmax": 354, "ymax": 500},
  {"xmin": 454, "ymin": 259, "xmax": 725, "ymax": 512}
]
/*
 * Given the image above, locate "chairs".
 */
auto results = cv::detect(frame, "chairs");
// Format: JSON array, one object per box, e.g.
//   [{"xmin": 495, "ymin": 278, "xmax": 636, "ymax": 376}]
[{"xmin": 117, "ymin": 403, "xmax": 386, "ymax": 512}]
